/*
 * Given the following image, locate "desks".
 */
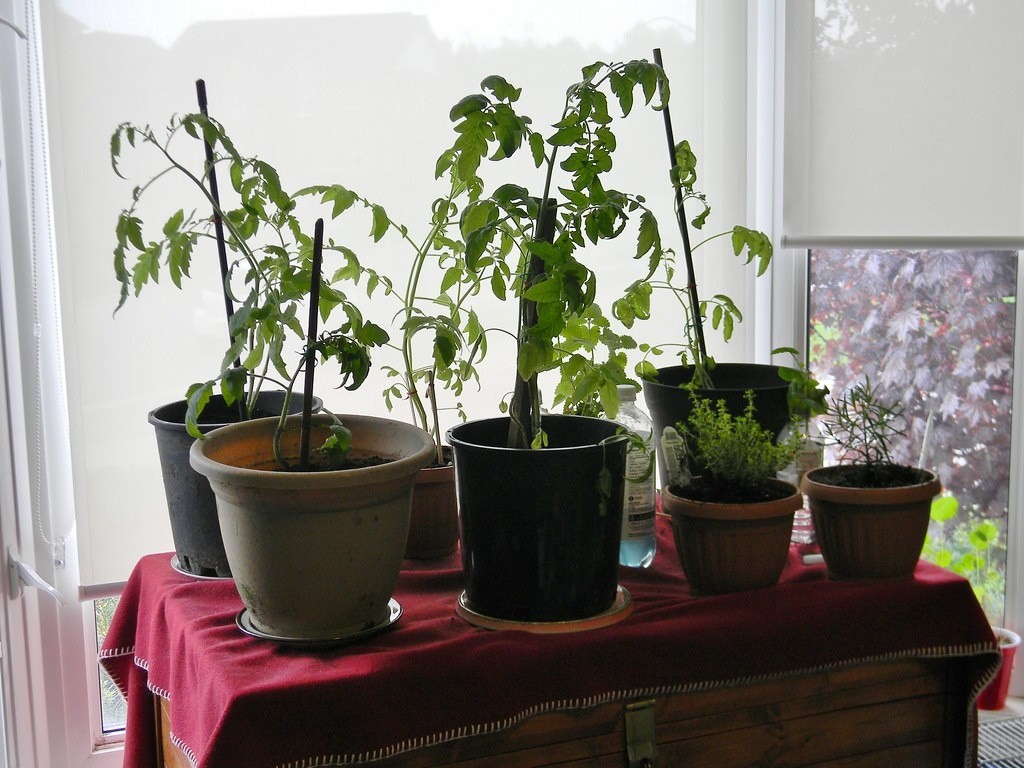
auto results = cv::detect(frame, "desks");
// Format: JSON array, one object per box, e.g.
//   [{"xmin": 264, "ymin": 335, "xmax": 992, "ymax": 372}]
[{"xmin": 93, "ymin": 490, "xmax": 1001, "ymax": 768}]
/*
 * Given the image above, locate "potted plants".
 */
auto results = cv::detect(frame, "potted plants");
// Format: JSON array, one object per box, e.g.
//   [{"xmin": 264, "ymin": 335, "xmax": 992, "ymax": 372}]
[
  {"xmin": 111, "ymin": 48, "xmax": 942, "ymax": 651},
  {"xmin": 918, "ymin": 492, "xmax": 1020, "ymax": 711}
]
